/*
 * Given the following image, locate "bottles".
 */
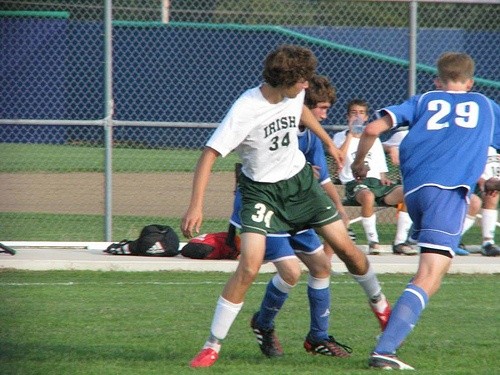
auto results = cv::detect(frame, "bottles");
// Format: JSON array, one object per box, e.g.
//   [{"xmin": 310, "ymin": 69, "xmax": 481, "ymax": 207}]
[{"xmin": 353, "ymin": 118, "xmax": 363, "ymax": 133}]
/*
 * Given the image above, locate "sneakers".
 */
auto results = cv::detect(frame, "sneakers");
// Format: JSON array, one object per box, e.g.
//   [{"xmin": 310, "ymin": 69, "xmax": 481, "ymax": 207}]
[
  {"xmin": 369, "ymin": 241, "xmax": 379, "ymax": 254},
  {"xmin": 251, "ymin": 311, "xmax": 282, "ymax": 356},
  {"xmin": 304, "ymin": 330, "xmax": 353, "ymax": 358},
  {"xmin": 368, "ymin": 352, "xmax": 416, "ymax": 371},
  {"xmin": 189, "ymin": 347, "xmax": 219, "ymax": 368},
  {"xmin": 392, "ymin": 240, "xmax": 416, "ymax": 255},
  {"xmin": 370, "ymin": 298, "xmax": 392, "ymax": 330},
  {"xmin": 482, "ymin": 243, "xmax": 500, "ymax": 256},
  {"xmin": 457, "ymin": 242, "xmax": 470, "ymax": 255}
]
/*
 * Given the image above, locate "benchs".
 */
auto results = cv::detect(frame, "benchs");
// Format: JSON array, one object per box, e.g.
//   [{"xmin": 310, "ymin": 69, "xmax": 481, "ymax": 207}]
[{"xmin": 324, "ymin": 150, "xmax": 397, "ymax": 230}]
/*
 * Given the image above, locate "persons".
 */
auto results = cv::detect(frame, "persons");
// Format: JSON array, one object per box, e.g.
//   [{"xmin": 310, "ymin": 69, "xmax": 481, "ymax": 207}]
[
  {"xmin": 351, "ymin": 52, "xmax": 500, "ymax": 370},
  {"xmin": 180, "ymin": 44, "xmax": 500, "ymax": 370}
]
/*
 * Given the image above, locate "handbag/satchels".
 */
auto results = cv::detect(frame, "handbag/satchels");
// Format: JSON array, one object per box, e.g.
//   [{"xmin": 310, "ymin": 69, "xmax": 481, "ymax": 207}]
[
  {"xmin": 104, "ymin": 225, "xmax": 180, "ymax": 255},
  {"xmin": 181, "ymin": 232, "xmax": 241, "ymax": 259}
]
